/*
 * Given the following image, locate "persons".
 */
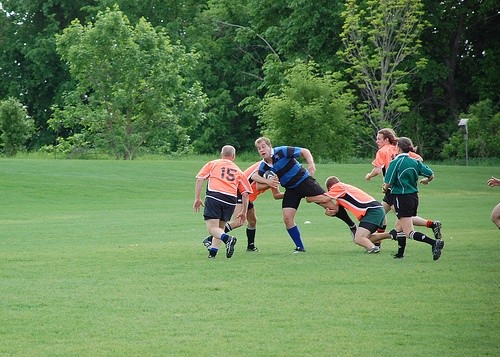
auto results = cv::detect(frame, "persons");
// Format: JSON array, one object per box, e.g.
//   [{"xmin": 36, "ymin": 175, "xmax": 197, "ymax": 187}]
[
  {"xmin": 366, "ymin": 128, "xmax": 442, "ymax": 246},
  {"xmin": 306, "ymin": 176, "xmax": 397, "ymax": 254},
  {"xmin": 193, "ymin": 145, "xmax": 253, "ymax": 258},
  {"xmin": 382, "ymin": 137, "xmax": 444, "ymax": 260},
  {"xmin": 255, "ymin": 138, "xmax": 358, "ymax": 253},
  {"xmin": 487, "ymin": 176, "xmax": 500, "ymax": 229},
  {"xmin": 203, "ymin": 160, "xmax": 285, "ymax": 252}
]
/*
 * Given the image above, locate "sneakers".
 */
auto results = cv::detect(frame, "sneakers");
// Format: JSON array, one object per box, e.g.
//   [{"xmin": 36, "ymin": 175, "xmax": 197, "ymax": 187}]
[
  {"xmin": 207, "ymin": 253, "xmax": 216, "ymax": 258},
  {"xmin": 431, "ymin": 239, "xmax": 444, "ymax": 260},
  {"xmin": 247, "ymin": 246, "xmax": 258, "ymax": 252},
  {"xmin": 202, "ymin": 238, "xmax": 211, "ymax": 251},
  {"xmin": 432, "ymin": 221, "xmax": 442, "ymax": 240},
  {"xmin": 365, "ymin": 246, "xmax": 379, "ymax": 254},
  {"xmin": 224, "ymin": 236, "xmax": 237, "ymax": 258},
  {"xmin": 390, "ymin": 229, "xmax": 398, "ymax": 242}
]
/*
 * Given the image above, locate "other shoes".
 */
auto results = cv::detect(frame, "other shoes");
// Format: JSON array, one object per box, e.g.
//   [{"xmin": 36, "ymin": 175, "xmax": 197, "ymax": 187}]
[
  {"xmin": 350, "ymin": 228, "xmax": 357, "ymax": 243},
  {"xmin": 293, "ymin": 247, "xmax": 306, "ymax": 253}
]
